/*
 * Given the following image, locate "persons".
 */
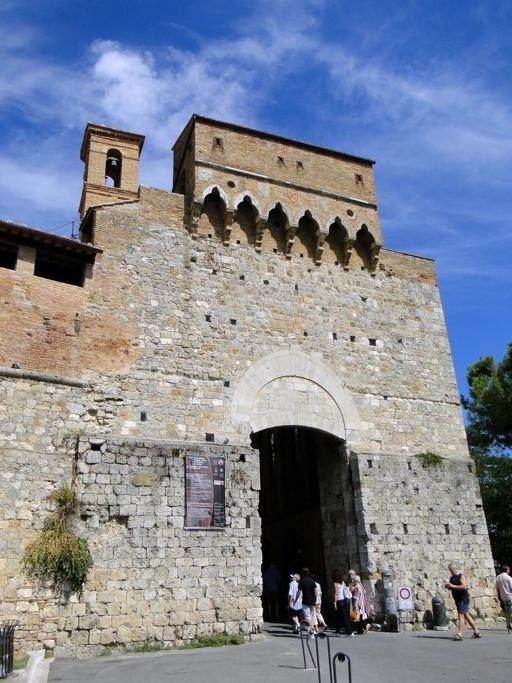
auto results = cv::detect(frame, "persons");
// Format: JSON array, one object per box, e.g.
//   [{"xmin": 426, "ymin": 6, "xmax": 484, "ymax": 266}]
[
  {"xmin": 494, "ymin": 564, "xmax": 512, "ymax": 629},
  {"xmin": 444, "ymin": 562, "xmax": 482, "ymax": 641},
  {"xmin": 286, "ymin": 567, "xmax": 372, "ymax": 638}
]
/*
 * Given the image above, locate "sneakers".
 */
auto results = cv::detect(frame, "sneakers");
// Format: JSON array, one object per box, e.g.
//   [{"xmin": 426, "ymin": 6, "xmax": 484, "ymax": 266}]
[{"xmin": 294, "ymin": 624, "xmax": 372, "ymax": 638}]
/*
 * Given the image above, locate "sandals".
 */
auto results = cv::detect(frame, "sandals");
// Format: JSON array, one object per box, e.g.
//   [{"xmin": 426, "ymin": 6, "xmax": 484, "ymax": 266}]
[
  {"xmin": 471, "ymin": 633, "xmax": 482, "ymax": 638},
  {"xmin": 453, "ymin": 633, "xmax": 463, "ymax": 640}
]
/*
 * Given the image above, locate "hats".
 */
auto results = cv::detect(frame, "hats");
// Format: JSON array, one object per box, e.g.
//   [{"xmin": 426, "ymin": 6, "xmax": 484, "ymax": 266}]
[{"xmin": 291, "ymin": 574, "xmax": 301, "ymax": 582}]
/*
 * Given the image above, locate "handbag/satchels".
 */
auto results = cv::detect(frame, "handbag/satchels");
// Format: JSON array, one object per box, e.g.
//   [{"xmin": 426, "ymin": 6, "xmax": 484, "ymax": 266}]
[{"xmin": 350, "ymin": 610, "xmax": 360, "ymax": 622}]
